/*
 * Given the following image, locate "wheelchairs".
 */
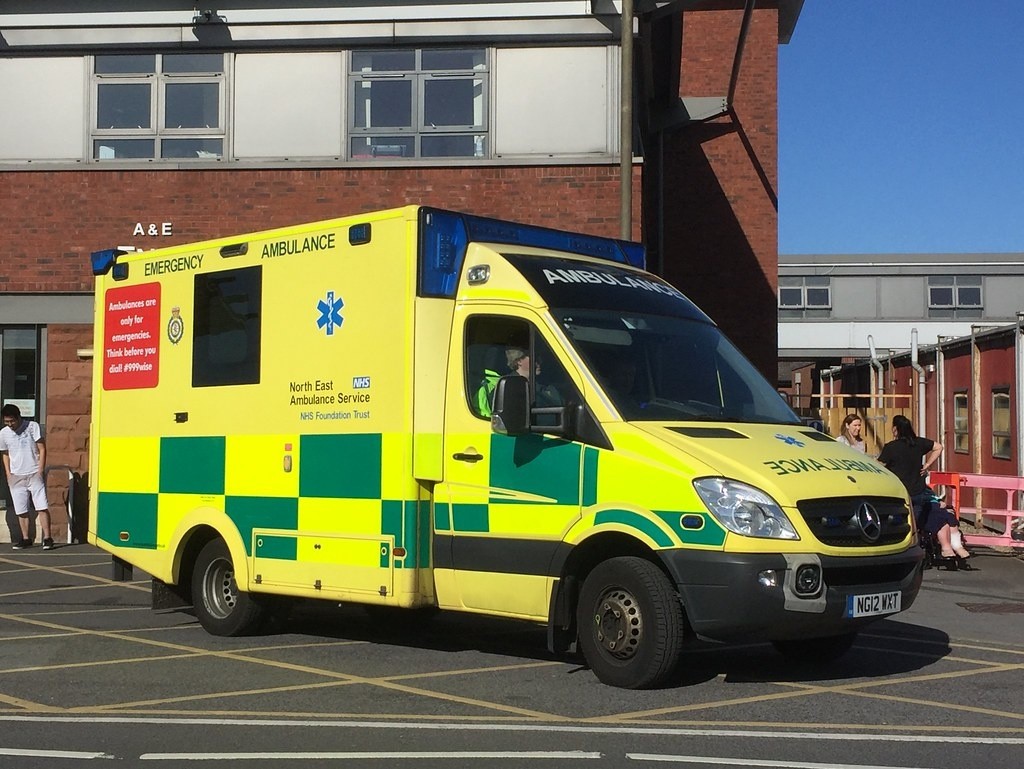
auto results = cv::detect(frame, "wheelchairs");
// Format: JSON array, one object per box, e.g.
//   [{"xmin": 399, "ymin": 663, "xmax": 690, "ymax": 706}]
[{"xmin": 915, "ymin": 505, "xmax": 974, "ymax": 567}]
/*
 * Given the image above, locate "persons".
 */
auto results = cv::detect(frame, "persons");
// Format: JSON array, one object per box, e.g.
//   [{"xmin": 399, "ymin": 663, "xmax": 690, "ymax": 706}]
[
  {"xmin": 474, "ymin": 330, "xmax": 548, "ymax": 416},
  {"xmin": 0, "ymin": 403, "xmax": 54, "ymax": 550},
  {"xmin": 876, "ymin": 412, "xmax": 971, "ymax": 560},
  {"xmin": 835, "ymin": 413, "xmax": 868, "ymax": 452}
]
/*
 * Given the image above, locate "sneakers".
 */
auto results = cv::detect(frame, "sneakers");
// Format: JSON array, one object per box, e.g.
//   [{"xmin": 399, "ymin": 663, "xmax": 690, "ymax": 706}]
[
  {"xmin": 43, "ymin": 537, "xmax": 54, "ymax": 550},
  {"xmin": 13, "ymin": 538, "xmax": 33, "ymax": 550}
]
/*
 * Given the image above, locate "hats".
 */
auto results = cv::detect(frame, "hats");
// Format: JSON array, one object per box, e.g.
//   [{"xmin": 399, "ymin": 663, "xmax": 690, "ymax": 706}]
[{"xmin": 505, "ymin": 330, "xmax": 545, "ymax": 366}]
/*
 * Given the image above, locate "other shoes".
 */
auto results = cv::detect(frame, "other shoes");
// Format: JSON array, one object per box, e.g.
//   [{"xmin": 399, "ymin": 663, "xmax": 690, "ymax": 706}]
[
  {"xmin": 942, "ymin": 548, "xmax": 956, "ymax": 557},
  {"xmin": 956, "ymin": 547, "xmax": 970, "ymax": 559}
]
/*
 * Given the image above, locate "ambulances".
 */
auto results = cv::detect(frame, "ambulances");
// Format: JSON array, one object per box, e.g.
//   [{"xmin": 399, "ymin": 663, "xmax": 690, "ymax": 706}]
[{"xmin": 86, "ymin": 202, "xmax": 933, "ymax": 688}]
